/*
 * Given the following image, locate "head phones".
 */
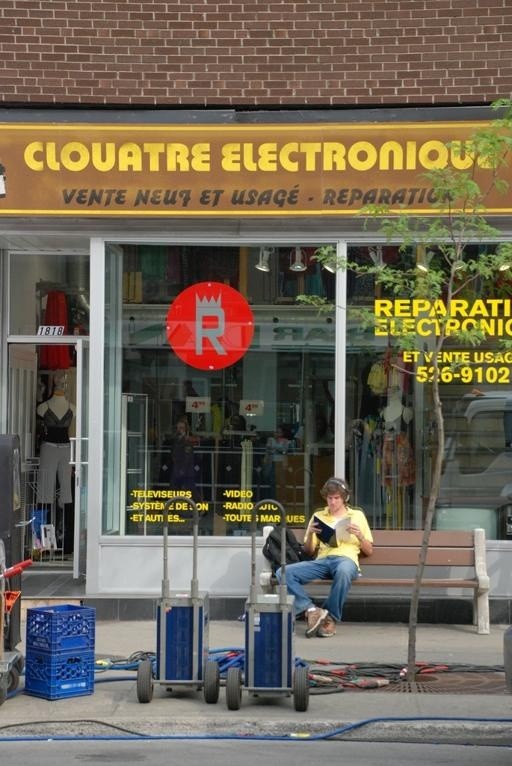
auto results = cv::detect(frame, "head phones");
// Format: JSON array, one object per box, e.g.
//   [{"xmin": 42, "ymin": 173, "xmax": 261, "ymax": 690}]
[{"xmin": 320, "ymin": 480, "xmax": 350, "ymax": 502}]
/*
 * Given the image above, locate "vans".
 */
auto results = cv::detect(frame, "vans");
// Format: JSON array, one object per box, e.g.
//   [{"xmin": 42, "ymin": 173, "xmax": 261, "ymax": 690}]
[{"xmin": 424, "ymin": 389, "xmax": 511, "ymax": 539}]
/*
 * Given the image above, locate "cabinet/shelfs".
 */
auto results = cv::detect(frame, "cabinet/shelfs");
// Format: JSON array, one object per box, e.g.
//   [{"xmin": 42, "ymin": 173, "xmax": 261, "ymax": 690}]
[{"xmin": 120, "ymin": 392, "xmax": 150, "ymax": 537}]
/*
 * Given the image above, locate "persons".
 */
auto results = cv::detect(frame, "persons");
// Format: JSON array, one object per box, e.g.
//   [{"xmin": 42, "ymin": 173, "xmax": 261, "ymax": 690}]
[
  {"xmin": 379, "ymin": 392, "xmax": 414, "ymax": 488},
  {"xmin": 162, "ymin": 410, "xmax": 289, "ymax": 493},
  {"xmin": 275, "ymin": 477, "xmax": 374, "ymax": 638},
  {"xmin": 34, "ymin": 371, "xmax": 76, "ymax": 505}
]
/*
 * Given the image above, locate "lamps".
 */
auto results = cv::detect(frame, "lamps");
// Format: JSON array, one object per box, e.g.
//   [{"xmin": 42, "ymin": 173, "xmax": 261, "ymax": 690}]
[
  {"xmin": 254, "ymin": 245, "xmax": 470, "ymax": 276},
  {"xmin": 494, "ymin": 244, "xmax": 512, "ymax": 272}
]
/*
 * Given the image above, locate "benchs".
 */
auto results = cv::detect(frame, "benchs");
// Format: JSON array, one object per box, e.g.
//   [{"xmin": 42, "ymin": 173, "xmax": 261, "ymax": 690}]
[{"xmin": 258, "ymin": 524, "xmax": 492, "ymax": 639}]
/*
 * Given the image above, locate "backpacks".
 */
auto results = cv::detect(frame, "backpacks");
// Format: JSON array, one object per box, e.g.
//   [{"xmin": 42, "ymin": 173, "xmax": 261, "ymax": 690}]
[{"xmin": 263, "ymin": 527, "xmax": 313, "ymax": 578}]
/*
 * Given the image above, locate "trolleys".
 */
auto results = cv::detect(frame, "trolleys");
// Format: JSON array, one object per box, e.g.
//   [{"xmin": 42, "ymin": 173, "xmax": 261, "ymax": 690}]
[
  {"xmin": 0, "ymin": 558, "xmax": 33, "ymax": 706},
  {"xmin": 136, "ymin": 497, "xmax": 310, "ymax": 711}
]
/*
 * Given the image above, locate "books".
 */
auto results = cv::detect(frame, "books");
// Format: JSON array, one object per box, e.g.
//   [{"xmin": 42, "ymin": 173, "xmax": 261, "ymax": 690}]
[{"xmin": 314, "ymin": 514, "xmax": 353, "ymax": 548}]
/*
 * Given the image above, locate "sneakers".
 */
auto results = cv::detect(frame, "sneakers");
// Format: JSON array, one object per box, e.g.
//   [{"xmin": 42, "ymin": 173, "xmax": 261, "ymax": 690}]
[{"xmin": 306, "ymin": 606, "xmax": 336, "ymax": 637}]
